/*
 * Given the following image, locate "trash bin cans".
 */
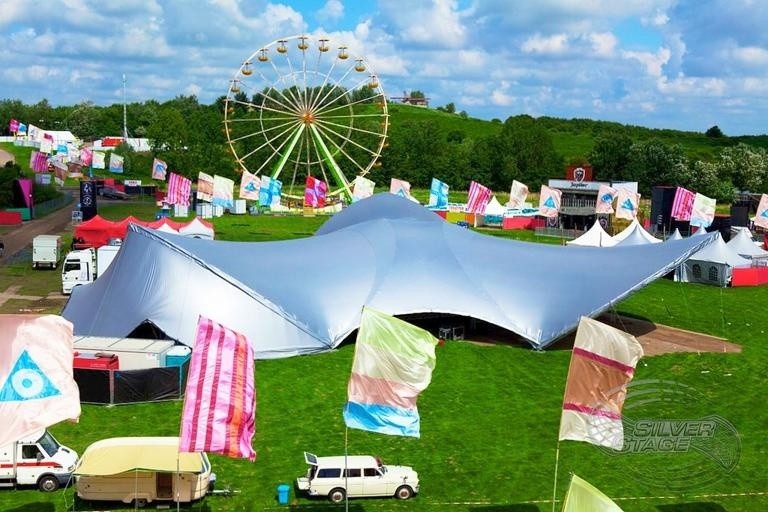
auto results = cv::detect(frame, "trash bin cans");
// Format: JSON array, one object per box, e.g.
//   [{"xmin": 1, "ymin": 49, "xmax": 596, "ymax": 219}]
[{"xmin": 278, "ymin": 485, "xmax": 289, "ymax": 503}]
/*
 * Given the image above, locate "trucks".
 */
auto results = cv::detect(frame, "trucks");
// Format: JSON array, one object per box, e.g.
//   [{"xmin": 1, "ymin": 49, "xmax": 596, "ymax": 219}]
[
  {"xmin": 61, "ymin": 245, "xmax": 121, "ymax": 293},
  {"xmin": 32, "ymin": 235, "xmax": 61, "ymax": 270},
  {"xmin": 0, "ymin": 427, "xmax": 79, "ymax": 492}
]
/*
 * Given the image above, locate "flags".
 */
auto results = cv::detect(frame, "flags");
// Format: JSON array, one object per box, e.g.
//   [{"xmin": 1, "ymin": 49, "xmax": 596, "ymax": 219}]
[
  {"xmin": 0, "ymin": 315, "xmax": 82, "ymax": 450},
  {"xmin": 178, "ymin": 314, "xmax": 257, "ymax": 462},
  {"xmin": 559, "ymin": 315, "xmax": 645, "ymax": 452},
  {"xmin": 342, "ymin": 306, "xmax": 439, "ymax": 439},
  {"xmin": 389, "ymin": 177, "xmax": 768, "ymax": 230},
  {"xmin": 151, "ymin": 158, "xmax": 378, "ymax": 209},
  {"xmin": 9, "ymin": 117, "xmax": 124, "ymax": 207}
]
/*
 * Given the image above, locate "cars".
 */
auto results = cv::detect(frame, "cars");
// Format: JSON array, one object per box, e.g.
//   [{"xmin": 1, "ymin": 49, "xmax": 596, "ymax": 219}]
[{"xmin": 297, "ymin": 451, "xmax": 420, "ymax": 503}]
